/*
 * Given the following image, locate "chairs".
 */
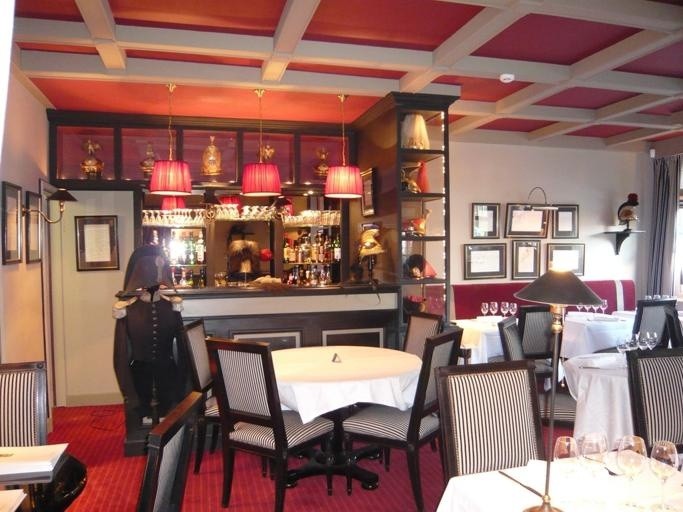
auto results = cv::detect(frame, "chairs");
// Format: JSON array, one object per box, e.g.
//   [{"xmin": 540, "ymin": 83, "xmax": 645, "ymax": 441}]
[
  {"xmin": 1, "ymin": 362, "xmax": 47, "ymax": 511},
  {"xmin": 137, "ymin": 391, "xmax": 201, "ymax": 512}
]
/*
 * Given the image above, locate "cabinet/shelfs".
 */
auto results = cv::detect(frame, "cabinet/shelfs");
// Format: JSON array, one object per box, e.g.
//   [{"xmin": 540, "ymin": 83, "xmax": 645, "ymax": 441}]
[{"xmin": 47, "ymin": 108, "xmax": 356, "ymax": 196}]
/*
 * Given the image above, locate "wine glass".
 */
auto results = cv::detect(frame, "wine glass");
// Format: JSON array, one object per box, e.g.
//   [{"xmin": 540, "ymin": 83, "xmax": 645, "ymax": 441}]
[
  {"xmin": 575, "ymin": 299, "xmax": 607, "ymax": 320},
  {"xmin": 612, "ymin": 331, "xmax": 658, "ymax": 367},
  {"xmin": 479, "ymin": 300, "xmax": 516, "ymax": 322},
  {"xmin": 551, "ymin": 430, "xmax": 678, "ymax": 510},
  {"xmin": 141, "ymin": 208, "xmax": 205, "ymax": 228},
  {"xmin": 205, "ymin": 203, "xmax": 278, "ymax": 221}
]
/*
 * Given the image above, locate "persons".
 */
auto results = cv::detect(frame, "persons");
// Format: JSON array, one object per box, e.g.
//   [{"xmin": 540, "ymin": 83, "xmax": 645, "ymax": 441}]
[{"xmin": 110, "ymin": 247, "xmax": 200, "ymax": 434}]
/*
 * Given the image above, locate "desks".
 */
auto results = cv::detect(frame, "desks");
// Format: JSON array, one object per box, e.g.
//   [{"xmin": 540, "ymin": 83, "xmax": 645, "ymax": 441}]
[{"xmin": 1, "ymin": 442, "xmax": 71, "ymax": 512}]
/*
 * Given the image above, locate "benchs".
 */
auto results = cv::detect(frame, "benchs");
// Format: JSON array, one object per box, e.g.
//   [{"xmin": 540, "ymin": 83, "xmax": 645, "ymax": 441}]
[{"xmin": 422, "ymin": 279, "xmax": 635, "ymax": 319}]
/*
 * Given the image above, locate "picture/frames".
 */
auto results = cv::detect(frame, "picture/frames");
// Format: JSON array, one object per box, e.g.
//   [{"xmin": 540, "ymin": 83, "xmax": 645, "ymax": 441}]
[
  {"xmin": 2, "ymin": 180, "xmax": 42, "ymax": 265},
  {"xmin": 74, "ymin": 216, "xmax": 119, "ymax": 271},
  {"xmin": 464, "ymin": 202, "xmax": 585, "ymax": 279}
]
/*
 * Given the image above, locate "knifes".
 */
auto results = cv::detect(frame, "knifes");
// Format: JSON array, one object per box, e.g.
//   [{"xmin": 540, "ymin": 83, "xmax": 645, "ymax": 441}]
[{"xmin": 578, "ymin": 366, "xmax": 615, "ymax": 372}]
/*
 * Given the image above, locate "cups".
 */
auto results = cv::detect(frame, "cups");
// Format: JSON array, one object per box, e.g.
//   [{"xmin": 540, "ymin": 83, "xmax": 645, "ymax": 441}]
[
  {"xmin": 642, "ymin": 294, "xmax": 677, "ymax": 300},
  {"xmin": 213, "ymin": 271, "xmax": 227, "ymax": 289},
  {"xmin": 283, "ymin": 209, "xmax": 340, "ymax": 226}
]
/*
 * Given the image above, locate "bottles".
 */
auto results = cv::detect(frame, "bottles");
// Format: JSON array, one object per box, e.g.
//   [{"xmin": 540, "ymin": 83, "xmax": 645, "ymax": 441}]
[
  {"xmin": 148, "ymin": 228, "xmax": 205, "ymax": 289},
  {"xmin": 281, "ymin": 231, "xmax": 341, "ymax": 286}
]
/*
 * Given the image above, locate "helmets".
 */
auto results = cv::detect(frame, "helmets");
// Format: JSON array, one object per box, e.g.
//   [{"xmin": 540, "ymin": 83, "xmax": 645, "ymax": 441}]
[{"xmin": 119, "ymin": 245, "xmax": 178, "ymax": 296}]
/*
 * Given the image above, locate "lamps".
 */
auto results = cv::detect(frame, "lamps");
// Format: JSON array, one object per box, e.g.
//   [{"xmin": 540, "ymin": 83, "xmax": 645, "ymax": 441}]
[
  {"xmin": 241, "ymin": 89, "xmax": 283, "ymax": 198},
  {"xmin": 22, "ymin": 188, "xmax": 78, "ymax": 224},
  {"xmin": 148, "ymin": 83, "xmax": 193, "ymax": 196},
  {"xmin": 513, "ymin": 270, "xmax": 603, "ymax": 512},
  {"xmin": 323, "ymin": 95, "xmax": 364, "ymax": 199}
]
